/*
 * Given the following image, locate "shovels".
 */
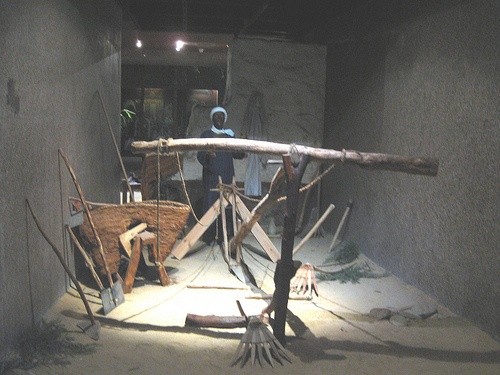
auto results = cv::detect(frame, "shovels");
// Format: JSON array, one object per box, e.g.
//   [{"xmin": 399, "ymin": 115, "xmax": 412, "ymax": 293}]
[
  {"xmin": 58, "ymin": 150, "xmax": 127, "ymax": 318},
  {"xmin": 23, "ymin": 197, "xmax": 102, "ymax": 342}
]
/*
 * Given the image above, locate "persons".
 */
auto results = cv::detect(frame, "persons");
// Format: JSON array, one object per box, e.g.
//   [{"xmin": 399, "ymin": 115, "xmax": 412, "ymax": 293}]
[{"xmin": 197, "ymin": 106, "xmax": 246, "ymax": 245}]
